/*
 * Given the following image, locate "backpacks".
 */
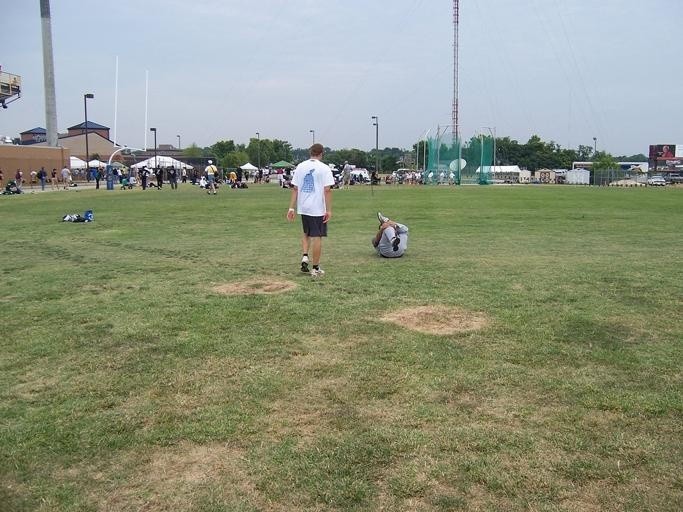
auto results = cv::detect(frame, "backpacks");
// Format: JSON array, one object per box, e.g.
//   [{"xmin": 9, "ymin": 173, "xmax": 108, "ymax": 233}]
[
  {"xmin": 16, "ymin": 173, "xmax": 20, "ymax": 179},
  {"xmin": 37, "ymin": 171, "xmax": 41, "ymax": 179}
]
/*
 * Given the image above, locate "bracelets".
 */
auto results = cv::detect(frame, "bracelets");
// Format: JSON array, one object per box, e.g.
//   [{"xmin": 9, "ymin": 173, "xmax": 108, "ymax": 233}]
[{"xmin": 288, "ymin": 208, "xmax": 294, "ymax": 211}]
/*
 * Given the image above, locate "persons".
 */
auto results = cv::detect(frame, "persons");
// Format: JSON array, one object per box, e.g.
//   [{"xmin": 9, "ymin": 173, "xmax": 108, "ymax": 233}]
[
  {"xmin": 371, "ymin": 211, "xmax": 408, "ymax": 258},
  {"xmin": 661, "ymin": 145, "xmax": 673, "ymax": 157},
  {"xmin": 285, "ymin": 144, "xmax": 334, "ymax": 277}
]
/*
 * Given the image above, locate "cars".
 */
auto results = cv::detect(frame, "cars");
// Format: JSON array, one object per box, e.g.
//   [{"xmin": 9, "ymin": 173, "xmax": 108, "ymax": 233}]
[
  {"xmin": 663, "ymin": 172, "xmax": 682, "ymax": 184},
  {"xmin": 648, "ymin": 175, "xmax": 666, "ymax": 186}
]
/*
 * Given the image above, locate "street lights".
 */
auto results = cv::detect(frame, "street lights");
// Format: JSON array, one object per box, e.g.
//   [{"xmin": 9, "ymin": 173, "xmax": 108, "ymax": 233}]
[
  {"xmin": 176, "ymin": 135, "xmax": 180, "ymax": 150},
  {"xmin": 372, "ymin": 116, "xmax": 379, "ymax": 177},
  {"xmin": 150, "ymin": 127, "xmax": 157, "ymax": 173},
  {"xmin": 84, "ymin": 94, "xmax": 95, "ymax": 182},
  {"xmin": 311, "ymin": 127, "xmax": 315, "ymax": 146},
  {"xmin": 256, "ymin": 131, "xmax": 261, "ymax": 169}
]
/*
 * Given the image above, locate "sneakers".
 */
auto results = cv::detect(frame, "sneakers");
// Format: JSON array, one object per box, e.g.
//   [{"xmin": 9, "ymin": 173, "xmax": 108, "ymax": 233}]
[
  {"xmin": 311, "ymin": 267, "xmax": 325, "ymax": 276},
  {"xmin": 392, "ymin": 237, "xmax": 400, "ymax": 251},
  {"xmin": 377, "ymin": 212, "xmax": 389, "ymax": 224},
  {"xmin": 301, "ymin": 256, "xmax": 310, "ymax": 272}
]
